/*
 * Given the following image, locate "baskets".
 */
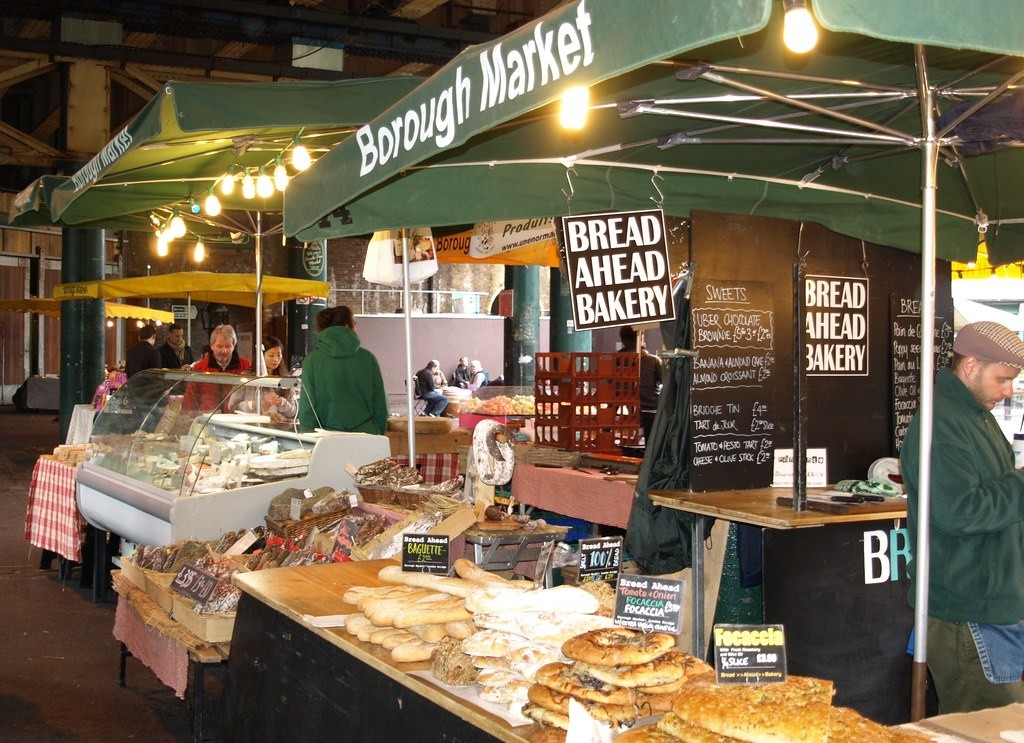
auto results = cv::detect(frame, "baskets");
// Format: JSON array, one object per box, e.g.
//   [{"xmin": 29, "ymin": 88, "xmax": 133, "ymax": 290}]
[
  {"xmin": 395, "ymin": 490, "xmax": 451, "ymax": 510},
  {"xmin": 355, "ymin": 482, "xmax": 400, "ymax": 506},
  {"xmin": 263, "ymin": 511, "xmax": 349, "ymax": 543}
]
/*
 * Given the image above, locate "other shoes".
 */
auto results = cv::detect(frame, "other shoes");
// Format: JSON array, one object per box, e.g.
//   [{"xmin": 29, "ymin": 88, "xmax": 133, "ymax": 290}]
[
  {"xmin": 428, "ymin": 413, "xmax": 436, "ymax": 417},
  {"xmin": 421, "ymin": 412, "xmax": 427, "ymax": 416}
]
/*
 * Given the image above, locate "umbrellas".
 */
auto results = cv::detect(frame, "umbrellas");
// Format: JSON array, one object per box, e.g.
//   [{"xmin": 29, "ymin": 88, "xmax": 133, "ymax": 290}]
[
  {"xmin": 53, "ymin": 271, "xmax": 329, "ymax": 346},
  {"xmin": 282, "ymin": 0, "xmax": 1024, "ymax": 721},
  {"xmin": 0, "ymin": 296, "xmax": 175, "ymax": 323},
  {"xmin": 8, "ymin": 75, "xmax": 428, "ymax": 414}
]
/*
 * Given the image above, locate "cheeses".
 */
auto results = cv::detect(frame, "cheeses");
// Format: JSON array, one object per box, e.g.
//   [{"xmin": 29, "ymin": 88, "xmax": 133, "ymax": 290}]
[{"xmin": 146, "ymin": 423, "xmax": 311, "ymax": 493}]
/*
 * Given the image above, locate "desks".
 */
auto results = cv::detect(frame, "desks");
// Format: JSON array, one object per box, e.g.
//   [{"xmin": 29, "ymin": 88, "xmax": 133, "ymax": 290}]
[
  {"xmin": 442, "ymin": 389, "xmax": 472, "ymax": 418},
  {"xmin": 215, "ymin": 558, "xmax": 970, "ymax": 743},
  {"xmin": 515, "ymin": 463, "xmax": 639, "ymax": 538},
  {"xmin": 385, "ymin": 429, "xmax": 471, "ymax": 484},
  {"xmin": 648, "ymin": 485, "xmax": 909, "ymax": 660},
  {"xmin": 459, "ymin": 413, "xmax": 535, "ymax": 432}
]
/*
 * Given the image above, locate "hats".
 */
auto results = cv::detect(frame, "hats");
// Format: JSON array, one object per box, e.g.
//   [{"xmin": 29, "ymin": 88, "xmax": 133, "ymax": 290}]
[{"xmin": 955, "ymin": 322, "xmax": 1024, "ymax": 371}]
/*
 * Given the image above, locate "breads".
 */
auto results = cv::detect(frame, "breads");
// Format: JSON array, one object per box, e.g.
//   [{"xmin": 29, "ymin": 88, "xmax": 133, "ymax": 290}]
[{"xmin": 341, "ymin": 558, "xmax": 635, "ymax": 723}]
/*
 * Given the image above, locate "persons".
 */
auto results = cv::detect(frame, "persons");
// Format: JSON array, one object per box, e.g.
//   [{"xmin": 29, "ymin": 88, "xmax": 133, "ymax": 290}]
[
  {"xmin": 467, "ymin": 359, "xmax": 488, "ymax": 391},
  {"xmin": 432, "ymin": 359, "xmax": 447, "ymax": 386},
  {"xmin": 182, "ymin": 324, "xmax": 252, "ymax": 413},
  {"xmin": 227, "ymin": 335, "xmax": 297, "ymax": 418},
  {"xmin": 455, "ymin": 356, "xmax": 470, "ymax": 388},
  {"xmin": 158, "ymin": 324, "xmax": 194, "ymax": 371},
  {"xmin": 297, "ymin": 306, "xmax": 388, "ymax": 435},
  {"xmin": 415, "ymin": 361, "xmax": 448, "ymax": 417},
  {"xmin": 125, "ymin": 325, "xmax": 162, "ymax": 403},
  {"xmin": 899, "ymin": 321, "xmax": 1024, "ymax": 715},
  {"xmin": 616, "ymin": 325, "xmax": 662, "ymax": 441}
]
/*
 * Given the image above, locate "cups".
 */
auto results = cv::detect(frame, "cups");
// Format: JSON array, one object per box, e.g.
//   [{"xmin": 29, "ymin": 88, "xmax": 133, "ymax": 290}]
[{"xmin": 65, "ymin": 403, "xmax": 96, "ymax": 447}]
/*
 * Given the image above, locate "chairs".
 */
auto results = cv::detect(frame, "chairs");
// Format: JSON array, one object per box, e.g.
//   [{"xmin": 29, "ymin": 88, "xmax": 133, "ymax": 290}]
[{"xmin": 405, "ymin": 376, "xmax": 426, "ymax": 416}]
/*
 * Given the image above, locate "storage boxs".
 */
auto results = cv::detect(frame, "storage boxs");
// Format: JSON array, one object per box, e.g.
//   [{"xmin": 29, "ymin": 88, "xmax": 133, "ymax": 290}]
[{"xmin": 534, "ymin": 352, "xmax": 639, "ymax": 452}]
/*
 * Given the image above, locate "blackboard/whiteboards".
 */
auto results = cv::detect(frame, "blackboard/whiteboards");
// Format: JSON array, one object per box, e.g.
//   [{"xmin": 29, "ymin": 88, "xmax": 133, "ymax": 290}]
[
  {"xmin": 690, "ymin": 279, "xmax": 777, "ymax": 493},
  {"xmin": 890, "ymin": 291, "xmax": 955, "ymax": 457}
]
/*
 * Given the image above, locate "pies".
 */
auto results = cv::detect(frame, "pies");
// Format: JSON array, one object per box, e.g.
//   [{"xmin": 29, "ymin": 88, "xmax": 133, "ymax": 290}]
[{"xmin": 521, "ymin": 627, "xmax": 939, "ymax": 743}]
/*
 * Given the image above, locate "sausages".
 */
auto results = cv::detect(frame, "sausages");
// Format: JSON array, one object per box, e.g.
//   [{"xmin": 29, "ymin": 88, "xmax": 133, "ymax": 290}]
[
  {"xmin": 356, "ymin": 457, "xmax": 463, "ymax": 493},
  {"xmin": 134, "ymin": 527, "xmax": 334, "ymax": 613}
]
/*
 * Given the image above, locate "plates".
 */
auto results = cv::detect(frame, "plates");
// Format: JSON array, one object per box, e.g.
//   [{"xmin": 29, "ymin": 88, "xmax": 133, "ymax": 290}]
[{"xmin": 867, "ymin": 457, "xmax": 905, "ymax": 496}]
